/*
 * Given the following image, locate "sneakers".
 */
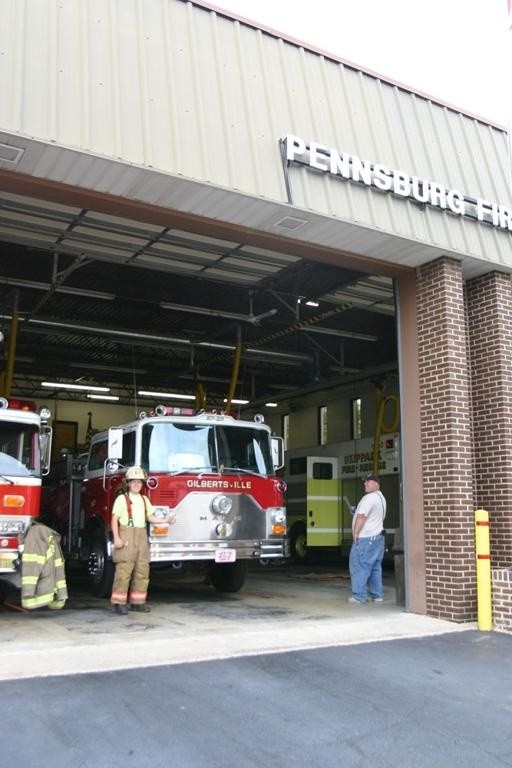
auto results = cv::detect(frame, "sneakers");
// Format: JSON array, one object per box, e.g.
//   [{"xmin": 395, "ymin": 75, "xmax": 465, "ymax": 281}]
[
  {"xmin": 374, "ymin": 597, "xmax": 383, "ymax": 602},
  {"xmin": 348, "ymin": 597, "xmax": 360, "ymax": 604},
  {"xmin": 130, "ymin": 604, "xmax": 151, "ymax": 613},
  {"xmin": 115, "ymin": 604, "xmax": 129, "ymax": 615}
]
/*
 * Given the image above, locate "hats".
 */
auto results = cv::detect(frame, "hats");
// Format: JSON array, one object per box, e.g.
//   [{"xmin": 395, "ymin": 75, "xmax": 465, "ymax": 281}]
[{"xmin": 362, "ymin": 472, "xmax": 379, "ymax": 481}]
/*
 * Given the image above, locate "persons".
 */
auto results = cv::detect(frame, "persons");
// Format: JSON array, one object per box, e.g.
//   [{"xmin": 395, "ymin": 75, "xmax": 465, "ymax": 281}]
[
  {"xmin": 346, "ymin": 473, "xmax": 387, "ymax": 603},
  {"xmin": 112, "ymin": 466, "xmax": 178, "ymax": 616}
]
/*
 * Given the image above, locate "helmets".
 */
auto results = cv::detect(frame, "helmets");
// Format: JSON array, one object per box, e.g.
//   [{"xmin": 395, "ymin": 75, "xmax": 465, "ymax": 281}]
[{"xmin": 124, "ymin": 465, "xmax": 147, "ymax": 480}]
